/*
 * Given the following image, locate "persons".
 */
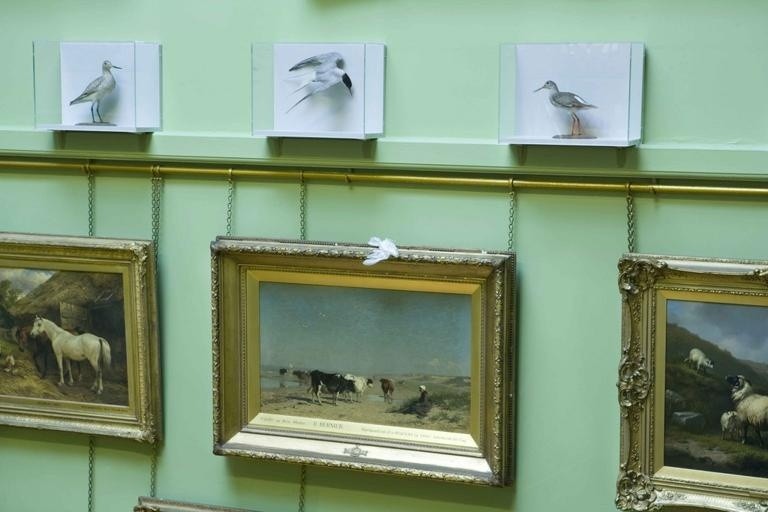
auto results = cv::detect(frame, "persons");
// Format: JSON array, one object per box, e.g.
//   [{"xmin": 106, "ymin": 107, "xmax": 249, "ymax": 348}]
[{"xmin": 410, "ymin": 383, "xmax": 432, "ymax": 417}]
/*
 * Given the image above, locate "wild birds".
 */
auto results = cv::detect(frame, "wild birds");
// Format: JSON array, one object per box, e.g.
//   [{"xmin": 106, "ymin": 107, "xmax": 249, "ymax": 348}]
[
  {"xmin": 534, "ymin": 80, "xmax": 599, "ymax": 137},
  {"xmin": 68, "ymin": 58, "xmax": 124, "ymax": 124},
  {"xmin": 284, "ymin": 52, "xmax": 353, "ymax": 114}
]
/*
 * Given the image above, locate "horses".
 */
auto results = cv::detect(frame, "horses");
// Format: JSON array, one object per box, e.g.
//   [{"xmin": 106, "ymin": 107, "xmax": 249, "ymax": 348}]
[
  {"xmin": 30, "ymin": 314, "xmax": 113, "ymax": 396},
  {"xmin": 14, "ymin": 324, "xmax": 87, "ymax": 383}
]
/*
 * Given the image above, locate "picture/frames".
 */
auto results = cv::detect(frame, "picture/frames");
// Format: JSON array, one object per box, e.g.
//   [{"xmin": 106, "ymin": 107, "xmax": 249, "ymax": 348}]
[
  {"xmin": 134, "ymin": 495, "xmax": 257, "ymax": 512},
  {"xmin": 210, "ymin": 235, "xmax": 517, "ymax": 489},
  {"xmin": 614, "ymin": 253, "xmax": 768, "ymax": 512},
  {"xmin": 1, "ymin": 232, "xmax": 164, "ymax": 447}
]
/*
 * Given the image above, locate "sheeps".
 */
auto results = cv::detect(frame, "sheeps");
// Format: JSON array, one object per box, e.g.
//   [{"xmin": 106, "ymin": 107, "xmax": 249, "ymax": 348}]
[
  {"xmin": 682, "ymin": 347, "xmax": 715, "ymax": 374},
  {"xmin": 719, "ymin": 374, "xmax": 768, "ymax": 448}
]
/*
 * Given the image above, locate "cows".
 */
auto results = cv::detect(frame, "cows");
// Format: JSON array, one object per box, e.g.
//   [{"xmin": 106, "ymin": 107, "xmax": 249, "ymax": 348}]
[
  {"xmin": 344, "ymin": 373, "xmax": 375, "ymax": 403},
  {"xmin": 279, "ymin": 368, "xmax": 288, "ymax": 376},
  {"xmin": 379, "ymin": 376, "xmax": 394, "ymax": 402},
  {"xmin": 292, "ymin": 370, "xmax": 310, "ymax": 387},
  {"xmin": 305, "ymin": 370, "xmax": 357, "ymax": 406}
]
